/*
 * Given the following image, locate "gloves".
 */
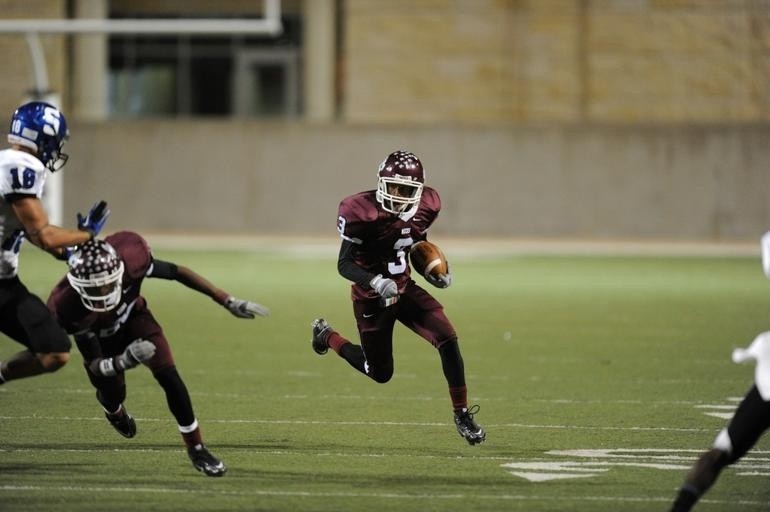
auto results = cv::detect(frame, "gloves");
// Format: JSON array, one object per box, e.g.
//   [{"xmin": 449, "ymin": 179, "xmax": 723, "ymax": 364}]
[
  {"xmin": 114, "ymin": 339, "xmax": 157, "ymax": 370},
  {"xmin": 62, "ymin": 245, "xmax": 78, "ymax": 258},
  {"xmin": 372, "ymin": 275, "xmax": 399, "ymax": 298},
  {"xmin": 76, "ymin": 198, "xmax": 110, "ymax": 235},
  {"xmin": 425, "ymin": 273, "xmax": 451, "ymax": 288},
  {"xmin": 224, "ymin": 297, "xmax": 267, "ymax": 318}
]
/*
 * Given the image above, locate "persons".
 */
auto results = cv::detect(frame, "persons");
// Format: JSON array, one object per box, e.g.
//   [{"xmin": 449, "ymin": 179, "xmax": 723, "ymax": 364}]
[
  {"xmin": 45, "ymin": 229, "xmax": 272, "ymax": 480},
  {"xmin": 309, "ymin": 150, "xmax": 488, "ymax": 448},
  {"xmin": 665, "ymin": 229, "xmax": 770, "ymax": 511},
  {"xmin": 0, "ymin": 100, "xmax": 114, "ymax": 387}
]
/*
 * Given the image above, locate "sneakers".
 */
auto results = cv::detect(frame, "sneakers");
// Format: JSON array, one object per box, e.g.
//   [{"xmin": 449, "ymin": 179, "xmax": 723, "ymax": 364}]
[
  {"xmin": 312, "ymin": 318, "xmax": 331, "ymax": 355},
  {"xmin": 187, "ymin": 442, "xmax": 226, "ymax": 476},
  {"xmin": 105, "ymin": 405, "xmax": 137, "ymax": 439},
  {"xmin": 452, "ymin": 406, "xmax": 486, "ymax": 444}
]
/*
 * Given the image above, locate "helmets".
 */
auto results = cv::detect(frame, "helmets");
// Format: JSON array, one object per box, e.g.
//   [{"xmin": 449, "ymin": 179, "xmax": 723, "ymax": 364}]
[
  {"xmin": 376, "ymin": 150, "xmax": 425, "ymax": 222},
  {"xmin": 8, "ymin": 101, "xmax": 72, "ymax": 172}
]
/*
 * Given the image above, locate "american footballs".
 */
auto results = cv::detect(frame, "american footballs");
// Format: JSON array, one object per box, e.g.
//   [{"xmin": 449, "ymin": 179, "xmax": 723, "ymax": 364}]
[{"xmin": 410, "ymin": 239, "xmax": 447, "ymax": 281}]
